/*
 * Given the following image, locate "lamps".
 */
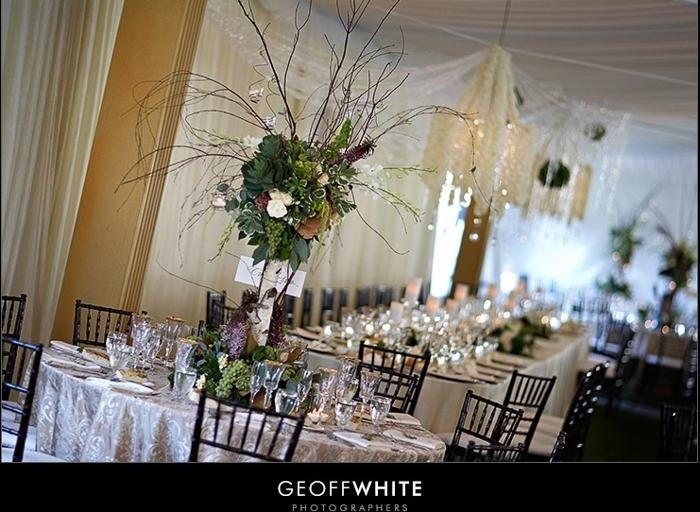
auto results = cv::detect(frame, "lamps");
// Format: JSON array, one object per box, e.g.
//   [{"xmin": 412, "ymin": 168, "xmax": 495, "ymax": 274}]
[{"xmin": 412, "ymin": 1, "xmax": 545, "ymax": 249}]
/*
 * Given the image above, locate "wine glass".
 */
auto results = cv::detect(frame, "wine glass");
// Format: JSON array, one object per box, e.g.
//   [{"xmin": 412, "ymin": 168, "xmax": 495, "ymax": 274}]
[
  {"xmin": 321, "ymin": 296, "xmax": 513, "ymax": 375},
  {"xmin": 104, "ymin": 312, "xmax": 392, "ymax": 429}
]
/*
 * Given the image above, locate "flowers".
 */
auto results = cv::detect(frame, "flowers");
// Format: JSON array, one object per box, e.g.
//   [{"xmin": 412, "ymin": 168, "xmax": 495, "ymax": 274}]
[
  {"xmin": 657, "ymin": 243, "xmax": 695, "ymax": 287},
  {"xmin": 184, "ymin": 82, "xmax": 441, "ymax": 273}
]
